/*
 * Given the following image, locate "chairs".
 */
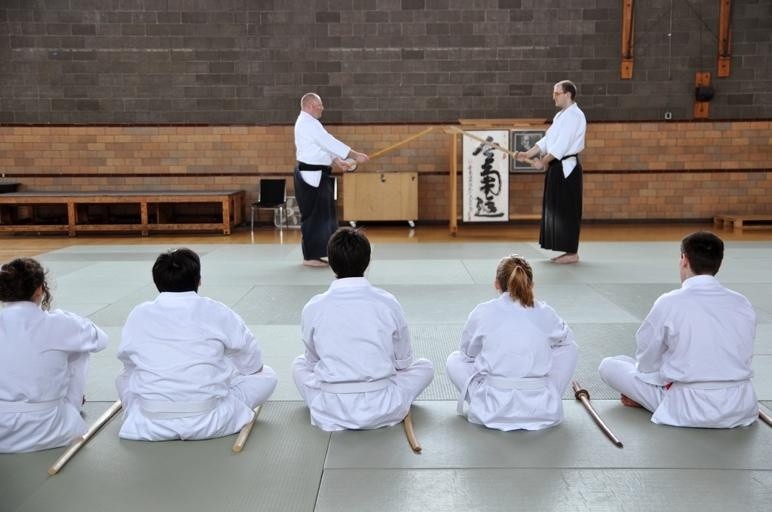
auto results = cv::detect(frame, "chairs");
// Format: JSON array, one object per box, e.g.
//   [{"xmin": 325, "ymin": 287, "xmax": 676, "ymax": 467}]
[{"xmin": 248, "ymin": 176, "xmax": 287, "ymax": 229}]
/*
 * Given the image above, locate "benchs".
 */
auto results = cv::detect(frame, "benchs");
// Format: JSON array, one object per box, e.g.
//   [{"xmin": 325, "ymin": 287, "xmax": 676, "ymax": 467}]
[{"xmin": 1, "ymin": 189, "xmax": 245, "ymax": 236}]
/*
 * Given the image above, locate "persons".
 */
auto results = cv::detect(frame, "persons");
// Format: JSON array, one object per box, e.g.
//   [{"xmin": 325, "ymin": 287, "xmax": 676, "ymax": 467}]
[
  {"xmin": 0, "ymin": 252, "xmax": 109, "ymax": 454},
  {"xmin": 293, "ymin": 92, "xmax": 369, "ymax": 268},
  {"xmin": 292, "ymin": 227, "xmax": 435, "ymax": 433},
  {"xmin": 114, "ymin": 247, "xmax": 277, "ymax": 444},
  {"xmin": 445, "ymin": 255, "xmax": 579, "ymax": 431},
  {"xmin": 596, "ymin": 231, "xmax": 760, "ymax": 430},
  {"xmin": 509, "ymin": 80, "xmax": 586, "ymax": 265}
]
